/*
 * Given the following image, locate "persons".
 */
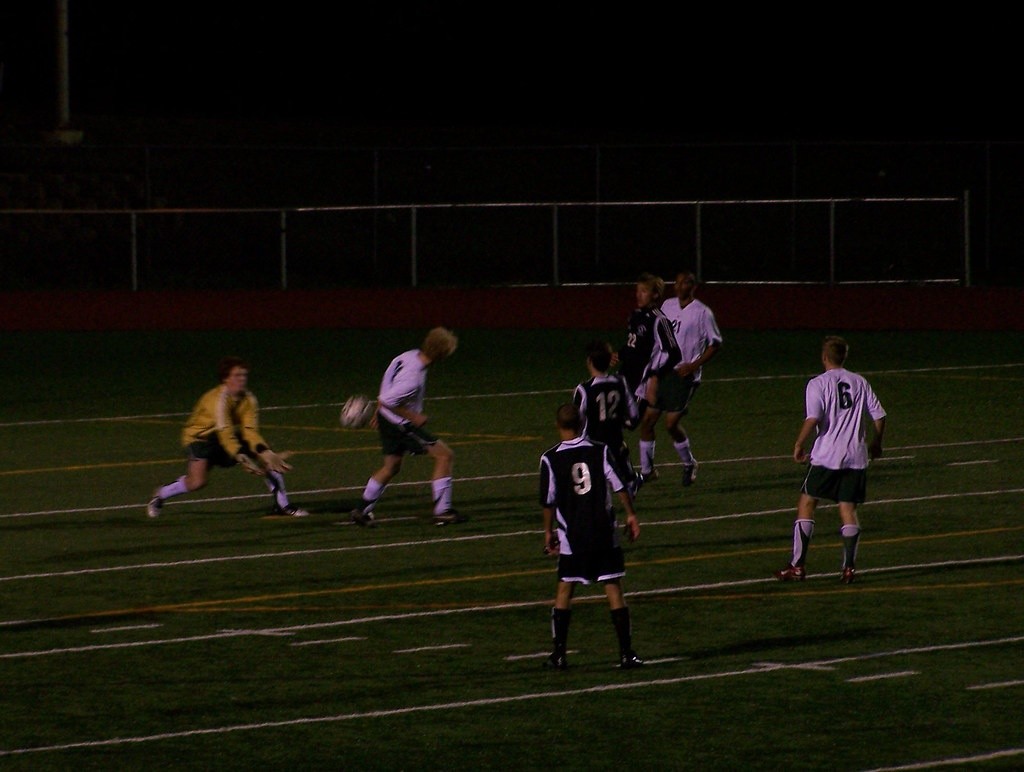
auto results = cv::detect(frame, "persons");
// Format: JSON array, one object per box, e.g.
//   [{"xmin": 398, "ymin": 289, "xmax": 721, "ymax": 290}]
[
  {"xmin": 538, "ymin": 272, "xmax": 723, "ymax": 670},
  {"xmin": 147, "ymin": 357, "xmax": 310, "ymax": 519},
  {"xmin": 772, "ymin": 336, "xmax": 887, "ymax": 584},
  {"xmin": 351, "ymin": 328, "xmax": 472, "ymax": 529}
]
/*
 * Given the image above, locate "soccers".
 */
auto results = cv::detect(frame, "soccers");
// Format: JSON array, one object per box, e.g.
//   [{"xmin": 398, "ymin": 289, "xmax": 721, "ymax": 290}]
[{"xmin": 339, "ymin": 394, "xmax": 374, "ymax": 429}]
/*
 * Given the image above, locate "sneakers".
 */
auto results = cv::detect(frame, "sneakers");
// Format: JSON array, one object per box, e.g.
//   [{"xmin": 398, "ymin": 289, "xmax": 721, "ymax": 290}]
[
  {"xmin": 840, "ymin": 567, "xmax": 855, "ymax": 584},
  {"xmin": 619, "ymin": 654, "xmax": 644, "ymax": 668},
  {"xmin": 431, "ymin": 508, "xmax": 468, "ymax": 527},
  {"xmin": 351, "ymin": 509, "xmax": 374, "ymax": 525},
  {"xmin": 682, "ymin": 458, "xmax": 698, "ymax": 486},
  {"xmin": 773, "ymin": 567, "xmax": 805, "ymax": 581},
  {"xmin": 636, "ymin": 468, "xmax": 659, "ymax": 483},
  {"xmin": 271, "ymin": 503, "xmax": 310, "ymax": 517},
  {"xmin": 147, "ymin": 484, "xmax": 166, "ymax": 519},
  {"xmin": 542, "ymin": 653, "xmax": 568, "ymax": 670}
]
